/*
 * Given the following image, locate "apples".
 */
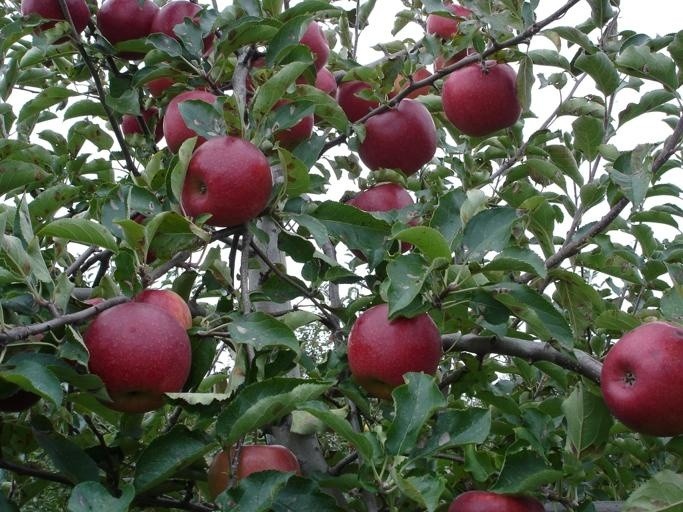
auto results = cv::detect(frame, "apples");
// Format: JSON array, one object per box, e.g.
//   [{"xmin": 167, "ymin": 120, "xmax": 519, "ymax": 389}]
[
  {"xmin": 21, "ymin": 0, "xmax": 88, "ymax": 44},
  {"xmin": 440, "ymin": 55, "xmax": 522, "ymax": 136},
  {"xmin": 447, "ymin": 490, "xmax": 545, "ymax": 512},
  {"xmin": 347, "ymin": 303, "xmax": 443, "ymax": 401},
  {"xmin": 356, "ymin": 97, "xmax": 438, "ymax": 182},
  {"xmin": 81, "ymin": 288, "xmax": 194, "ymax": 414},
  {"xmin": 97, "ymin": 0, "xmax": 488, "ymax": 159},
  {"xmin": 339, "ymin": 182, "xmax": 419, "ymax": 259},
  {"xmin": 599, "ymin": 321, "xmax": 683, "ymax": 436},
  {"xmin": 179, "ymin": 134, "xmax": 272, "ymax": 230},
  {"xmin": 209, "ymin": 444, "xmax": 300, "ymax": 502}
]
